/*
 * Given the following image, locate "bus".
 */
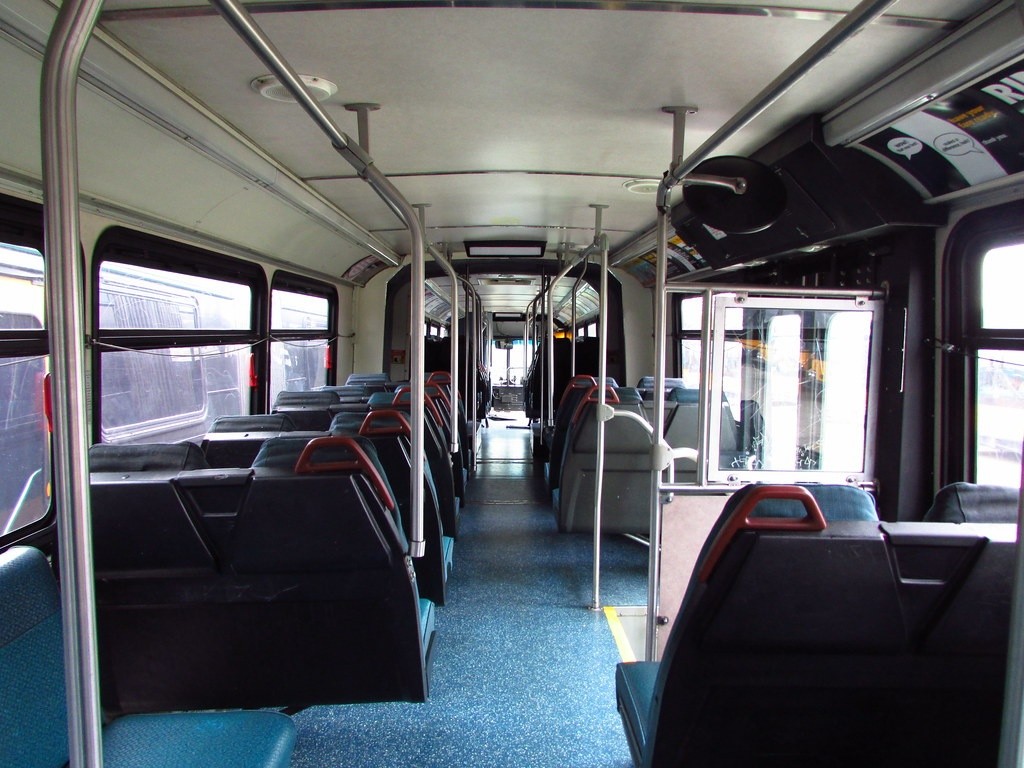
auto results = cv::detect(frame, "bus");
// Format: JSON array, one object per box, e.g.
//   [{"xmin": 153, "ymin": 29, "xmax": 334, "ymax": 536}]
[{"xmin": 0, "ymin": 240, "xmax": 328, "ymax": 537}]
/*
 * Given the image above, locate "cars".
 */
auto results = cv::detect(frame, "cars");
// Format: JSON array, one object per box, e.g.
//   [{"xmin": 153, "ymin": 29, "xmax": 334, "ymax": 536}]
[{"xmin": 736, "ymin": 308, "xmax": 836, "ymax": 396}]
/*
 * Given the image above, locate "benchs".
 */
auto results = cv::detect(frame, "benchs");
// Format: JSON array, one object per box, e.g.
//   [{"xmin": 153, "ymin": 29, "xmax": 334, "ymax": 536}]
[{"xmin": 0, "ymin": 336, "xmax": 1024, "ymax": 768}]
